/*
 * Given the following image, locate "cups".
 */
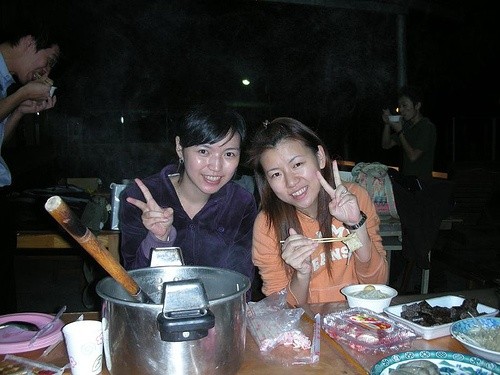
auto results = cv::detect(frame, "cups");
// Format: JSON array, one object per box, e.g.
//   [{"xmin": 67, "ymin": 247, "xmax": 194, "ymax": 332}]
[
  {"xmin": 62, "ymin": 319, "xmax": 103, "ymax": 375},
  {"xmin": 102, "ymin": 317, "xmax": 112, "ymax": 372}
]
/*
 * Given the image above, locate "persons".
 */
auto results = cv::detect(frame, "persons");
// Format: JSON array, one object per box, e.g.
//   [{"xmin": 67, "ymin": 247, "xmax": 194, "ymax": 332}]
[
  {"xmin": 383, "ymin": 87, "xmax": 442, "ymax": 287},
  {"xmin": 0, "ymin": 18, "xmax": 64, "ymax": 311},
  {"xmin": 249, "ymin": 117, "xmax": 389, "ymax": 306},
  {"xmin": 118, "ymin": 99, "xmax": 258, "ymax": 303}
]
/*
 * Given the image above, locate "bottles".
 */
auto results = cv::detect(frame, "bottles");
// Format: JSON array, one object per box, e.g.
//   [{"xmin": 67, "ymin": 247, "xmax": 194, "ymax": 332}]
[{"xmin": 110, "ymin": 178, "xmax": 131, "ymax": 230}]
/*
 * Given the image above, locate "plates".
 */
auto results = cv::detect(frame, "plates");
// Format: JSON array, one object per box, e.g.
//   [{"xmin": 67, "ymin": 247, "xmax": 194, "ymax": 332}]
[
  {"xmin": 370, "ymin": 349, "xmax": 500, "ymax": 375},
  {"xmin": 384, "ymin": 295, "xmax": 500, "ymax": 341}
]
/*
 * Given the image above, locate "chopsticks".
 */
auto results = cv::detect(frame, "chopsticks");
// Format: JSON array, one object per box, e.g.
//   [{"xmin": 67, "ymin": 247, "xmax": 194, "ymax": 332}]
[
  {"xmin": 35, "ymin": 72, "xmax": 53, "ymax": 86},
  {"xmin": 243, "ymin": 301, "xmax": 312, "ymax": 351},
  {"xmin": 279, "ymin": 237, "xmax": 344, "ymax": 246}
]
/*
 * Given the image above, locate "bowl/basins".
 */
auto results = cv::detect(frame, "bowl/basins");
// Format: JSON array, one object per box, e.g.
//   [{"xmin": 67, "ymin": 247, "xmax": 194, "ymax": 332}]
[
  {"xmin": 449, "ymin": 316, "xmax": 500, "ymax": 362},
  {"xmin": 339, "ymin": 283, "xmax": 398, "ymax": 313},
  {"xmin": 387, "ymin": 115, "xmax": 400, "ymax": 123},
  {"xmin": 49, "ymin": 87, "xmax": 57, "ymax": 98}
]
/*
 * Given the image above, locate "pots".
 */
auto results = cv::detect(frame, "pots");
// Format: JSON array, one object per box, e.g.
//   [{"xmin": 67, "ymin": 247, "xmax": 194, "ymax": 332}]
[{"xmin": 96, "ymin": 246, "xmax": 252, "ymax": 375}]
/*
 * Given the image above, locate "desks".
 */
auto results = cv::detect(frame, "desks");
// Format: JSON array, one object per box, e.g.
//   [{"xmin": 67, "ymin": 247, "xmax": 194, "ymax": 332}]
[{"xmin": 12, "ymin": 287, "xmax": 500, "ymax": 375}]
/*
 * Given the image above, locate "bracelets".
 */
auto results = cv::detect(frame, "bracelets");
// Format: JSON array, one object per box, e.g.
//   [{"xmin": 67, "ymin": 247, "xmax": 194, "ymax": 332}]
[
  {"xmin": 343, "ymin": 211, "xmax": 367, "ymax": 230},
  {"xmin": 396, "ymin": 130, "xmax": 403, "ymax": 135}
]
